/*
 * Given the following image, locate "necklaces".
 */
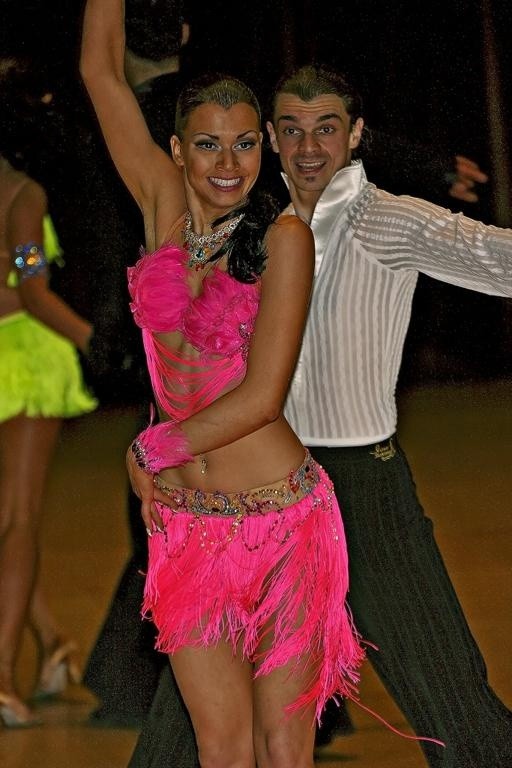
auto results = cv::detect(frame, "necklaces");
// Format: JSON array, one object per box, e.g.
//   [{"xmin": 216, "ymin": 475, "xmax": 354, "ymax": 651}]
[{"xmin": 178, "ymin": 214, "xmax": 249, "ymax": 269}]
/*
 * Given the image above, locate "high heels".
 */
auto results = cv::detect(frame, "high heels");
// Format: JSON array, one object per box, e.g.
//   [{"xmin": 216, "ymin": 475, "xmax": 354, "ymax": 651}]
[
  {"xmin": 23, "ymin": 637, "xmax": 83, "ymax": 705},
  {"xmin": 0, "ymin": 690, "xmax": 45, "ymax": 731}
]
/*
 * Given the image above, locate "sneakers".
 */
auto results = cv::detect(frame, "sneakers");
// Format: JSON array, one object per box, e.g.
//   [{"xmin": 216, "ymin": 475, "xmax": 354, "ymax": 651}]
[{"xmin": 313, "ymin": 692, "xmax": 357, "ymax": 749}]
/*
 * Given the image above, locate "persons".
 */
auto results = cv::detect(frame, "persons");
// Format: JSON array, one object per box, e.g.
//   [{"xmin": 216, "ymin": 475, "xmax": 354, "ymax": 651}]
[
  {"xmin": 73, "ymin": 0, "xmax": 361, "ymax": 768},
  {"xmin": 128, "ymin": 64, "xmax": 511, "ymax": 767},
  {"xmin": 68, "ymin": 0, "xmax": 489, "ymax": 749},
  {"xmin": 2, "ymin": 55, "xmax": 102, "ymax": 726}
]
